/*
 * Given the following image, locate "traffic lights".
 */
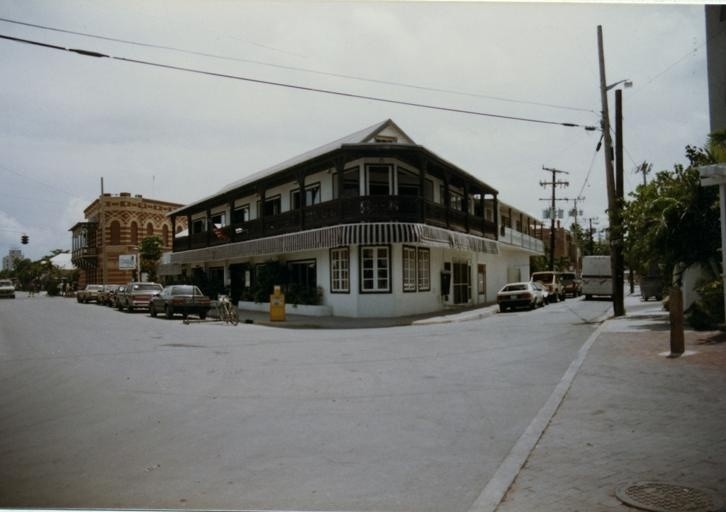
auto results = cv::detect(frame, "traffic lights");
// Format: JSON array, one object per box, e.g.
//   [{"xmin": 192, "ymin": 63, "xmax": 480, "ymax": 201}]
[{"xmin": 20, "ymin": 235, "xmax": 29, "ymax": 245}]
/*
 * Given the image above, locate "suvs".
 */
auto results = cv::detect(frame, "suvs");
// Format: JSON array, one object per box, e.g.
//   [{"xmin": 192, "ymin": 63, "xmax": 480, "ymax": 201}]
[{"xmin": 494, "ymin": 268, "xmax": 579, "ymax": 314}]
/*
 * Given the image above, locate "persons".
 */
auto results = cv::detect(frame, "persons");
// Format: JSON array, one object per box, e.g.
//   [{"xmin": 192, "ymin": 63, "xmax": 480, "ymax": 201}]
[{"xmin": 12, "ymin": 270, "xmax": 74, "ymax": 298}]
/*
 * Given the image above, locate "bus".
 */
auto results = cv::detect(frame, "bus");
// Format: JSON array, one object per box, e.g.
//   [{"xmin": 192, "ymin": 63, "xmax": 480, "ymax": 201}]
[{"xmin": 581, "ymin": 254, "xmax": 612, "ymax": 300}]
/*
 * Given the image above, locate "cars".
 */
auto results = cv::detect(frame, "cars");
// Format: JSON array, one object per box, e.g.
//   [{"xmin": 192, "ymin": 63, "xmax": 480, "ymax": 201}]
[
  {"xmin": 75, "ymin": 283, "xmax": 102, "ymax": 304},
  {"xmin": 1, "ymin": 275, "xmax": 16, "ymax": 300},
  {"xmin": 144, "ymin": 284, "xmax": 209, "ymax": 320},
  {"xmin": 96, "ymin": 281, "xmax": 163, "ymax": 314}
]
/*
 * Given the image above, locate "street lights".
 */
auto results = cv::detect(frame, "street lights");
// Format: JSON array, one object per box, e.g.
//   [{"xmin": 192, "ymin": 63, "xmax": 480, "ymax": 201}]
[{"xmin": 598, "ymin": 75, "xmax": 635, "ymax": 316}]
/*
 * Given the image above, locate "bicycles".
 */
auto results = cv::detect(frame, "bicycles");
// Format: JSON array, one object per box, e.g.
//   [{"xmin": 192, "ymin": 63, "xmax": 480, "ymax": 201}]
[{"xmin": 211, "ymin": 292, "xmax": 239, "ymax": 326}]
